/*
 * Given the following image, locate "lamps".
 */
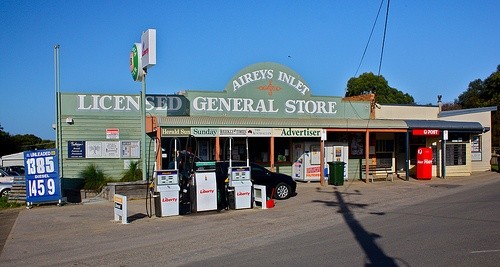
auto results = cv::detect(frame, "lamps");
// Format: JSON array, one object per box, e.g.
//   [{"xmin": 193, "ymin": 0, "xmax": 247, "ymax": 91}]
[
  {"xmin": 66, "ymin": 117, "xmax": 73, "ymax": 122},
  {"xmin": 483, "ymin": 127, "xmax": 491, "ymax": 132},
  {"xmin": 374, "ymin": 103, "xmax": 382, "ymax": 108}
]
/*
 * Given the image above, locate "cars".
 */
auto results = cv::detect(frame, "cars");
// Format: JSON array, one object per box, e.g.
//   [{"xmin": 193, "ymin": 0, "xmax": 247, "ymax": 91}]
[{"xmin": 0, "ymin": 166, "xmax": 26, "ymax": 197}]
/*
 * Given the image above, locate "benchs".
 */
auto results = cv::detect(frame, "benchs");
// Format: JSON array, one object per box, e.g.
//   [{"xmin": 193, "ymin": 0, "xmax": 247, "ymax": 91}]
[{"xmin": 361, "ymin": 165, "xmax": 397, "ymax": 183}]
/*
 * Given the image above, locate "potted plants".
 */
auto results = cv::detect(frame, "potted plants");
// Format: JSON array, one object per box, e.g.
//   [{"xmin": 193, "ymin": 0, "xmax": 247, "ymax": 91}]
[{"xmin": 78, "ymin": 163, "xmax": 110, "ymax": 200}]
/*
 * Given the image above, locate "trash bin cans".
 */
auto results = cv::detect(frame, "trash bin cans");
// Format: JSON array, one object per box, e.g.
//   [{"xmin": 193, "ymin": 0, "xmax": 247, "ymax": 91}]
[{"xmin": 327, "ymin": 161, "xmax": 346, "ymax": 186}]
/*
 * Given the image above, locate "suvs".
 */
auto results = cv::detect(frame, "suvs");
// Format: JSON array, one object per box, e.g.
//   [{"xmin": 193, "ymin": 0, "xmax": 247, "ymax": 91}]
[{"xmin": 182, "ymin": 160, "xmax": 297, "ymax": 200}]
